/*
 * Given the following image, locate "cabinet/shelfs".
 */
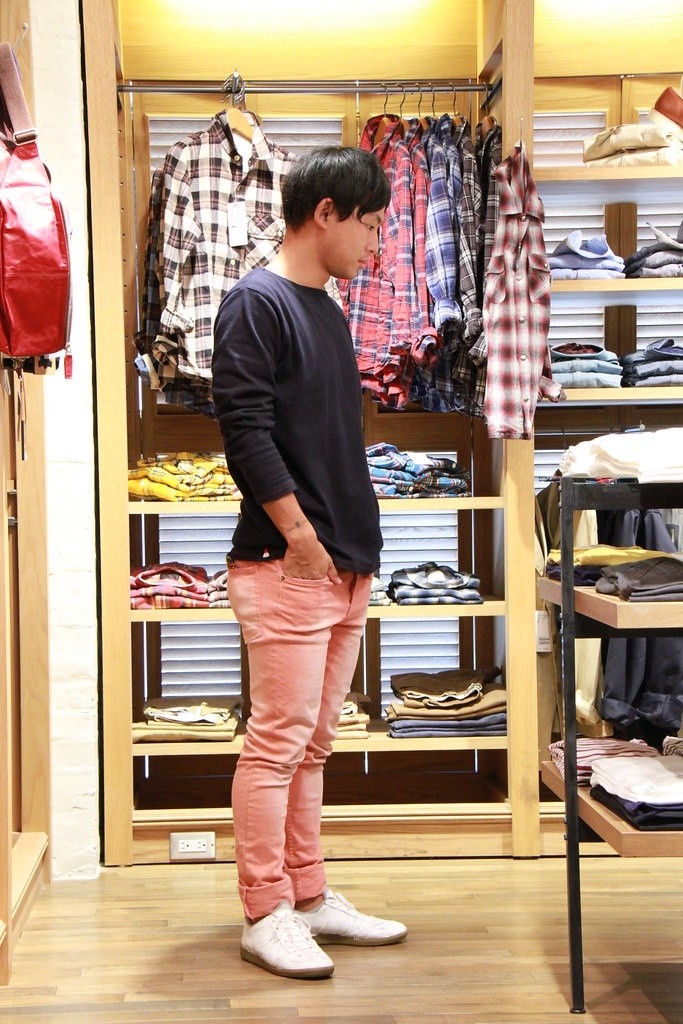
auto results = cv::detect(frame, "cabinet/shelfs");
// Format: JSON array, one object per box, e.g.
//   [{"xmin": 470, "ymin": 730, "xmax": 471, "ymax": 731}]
[
  {"xmin": 504, "ymin": 0, "xmax": 683, "ymax": 859},
  {"xmin": 82, "ymin": 1, "xmax": 524, "ymax": 863},
  {"xmin": 556, "ymin": 476, "xmax": 683, "ymax": 1014}
]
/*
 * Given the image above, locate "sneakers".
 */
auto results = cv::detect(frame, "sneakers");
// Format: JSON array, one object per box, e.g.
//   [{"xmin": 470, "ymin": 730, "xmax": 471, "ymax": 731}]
[
  {"xmin": 240, "ymin": 899, "xmax": 335, "ymax": 978},
  {"xmin": 292, "ymin": 885, "xmax": 408, "ymax": 946}
]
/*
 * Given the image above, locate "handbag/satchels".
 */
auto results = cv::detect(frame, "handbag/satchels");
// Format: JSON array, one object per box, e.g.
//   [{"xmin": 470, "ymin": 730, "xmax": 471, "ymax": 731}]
[{"xmin": 0, "ymin": 42, "xmax": 72, "ymax": 362}]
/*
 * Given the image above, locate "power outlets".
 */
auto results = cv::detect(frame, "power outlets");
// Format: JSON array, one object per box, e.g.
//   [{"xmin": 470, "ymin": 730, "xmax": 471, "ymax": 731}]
[{"xmin": 169, "ymin": 831, "xmax": 215, "ymax": 860}]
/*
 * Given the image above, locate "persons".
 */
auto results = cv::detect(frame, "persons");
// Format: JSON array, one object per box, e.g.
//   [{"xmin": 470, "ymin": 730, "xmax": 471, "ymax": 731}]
[{"xmin": 210, "ymin": 145, "xmax": 408, "ymax": 982}]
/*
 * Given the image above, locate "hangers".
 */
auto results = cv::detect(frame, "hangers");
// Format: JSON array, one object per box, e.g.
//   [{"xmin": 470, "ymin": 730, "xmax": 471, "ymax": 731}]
[
  {"xmin": 219, "ymin": 75, "xmax": 253, "ymax": 141},
  {"xmin": 374, "ymin": 83, "xmax": 494, "ymax": 147}
]
